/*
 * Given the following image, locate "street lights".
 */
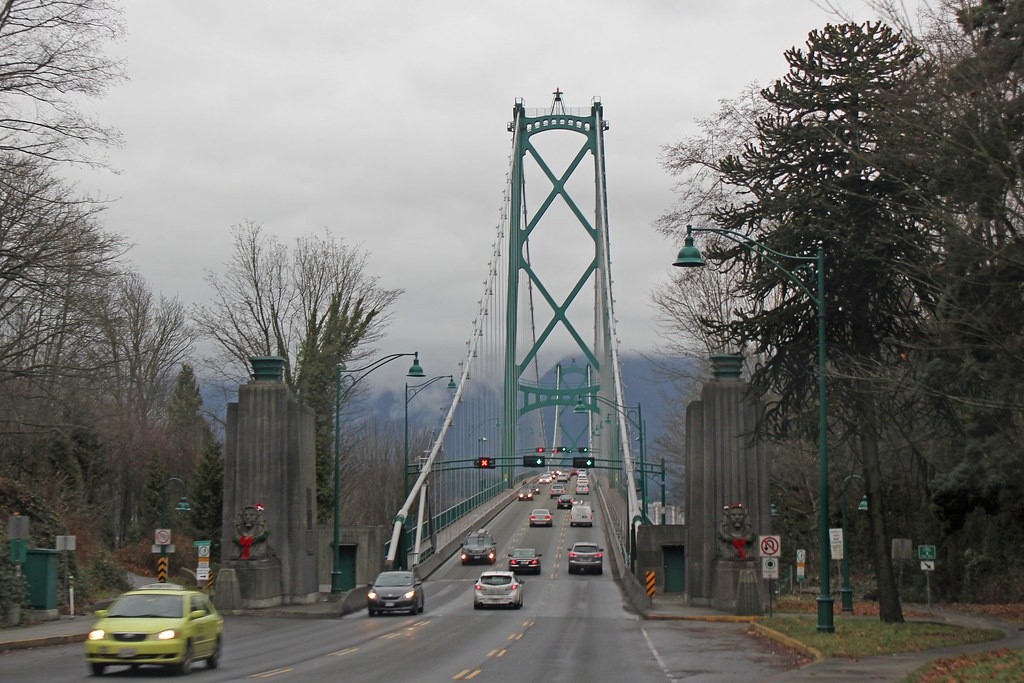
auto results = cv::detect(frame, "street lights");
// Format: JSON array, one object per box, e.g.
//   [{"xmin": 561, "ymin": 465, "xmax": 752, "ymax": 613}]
[
  {"xmin": 153, "ymin": 475, "xmax": 191, "ymax": 584},
  {"xmin": 402, "ymin": 374, "xmax": 458, "ymax": 571},
  {"xmin": 465, "ymin": 418, "xmax": 544, "ymax": 498},
  {"xmin": 673, "ymin": 223, "xmax": 836, "ymax": 636},
  {"xmin": 574, "ymin": 392, "xmax": 648, "ymax": 529},
  {"xmin": 327, "ymin": 351, "xmax": 425, "ymax": 595}
]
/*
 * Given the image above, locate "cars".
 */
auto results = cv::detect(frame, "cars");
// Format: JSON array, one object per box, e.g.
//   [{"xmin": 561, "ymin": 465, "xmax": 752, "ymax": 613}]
[
  {"xmin": 529, "ymin": 509, "xmax": 553, "ymax": 528},
  {"xmin": 508, "ymin": 548, "xmax": 543, "ymax": 573},
  {"xmin": 556, "ymin": 495, "xmax": 573, "ymax": 510},
  {"xmin": 473, "ymin": 571, "xmax": 524, "ymax": 609},
  {"xmin": 367, "ymin": 571, "xmax": 424, "ymax": 613},
  {"xmin": 460, "ymin": 531, "xmax": 496, "ymax": 563},
  {"xmin": 570, "ymin": 500, "xmax": 593, "ymax": 527},
  {"xmin": 567, "ymin": 542, "xmax": 605, "ymax": 575},
  {"xmin": 83, "ymin": 584, "xmax": 225, "ymax": 673},
  {"xmin": 517, "ymin": 467, "xmax": 589, "ymax": 501}
]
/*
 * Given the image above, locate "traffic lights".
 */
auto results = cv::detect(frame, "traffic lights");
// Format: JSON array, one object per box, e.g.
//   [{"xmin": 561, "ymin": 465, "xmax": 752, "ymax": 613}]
[
  {"xmin": 536, "ymin": 447, "xmax": 589, "ymax": 453},
  {"xmin": 523, "ymin": 455, "xmax": 545, "ymax": 467},
  {"xmin": 476, "ymin": 457, "xmax": 490, "ymax": 470},
  {"xmin": 572, "ymin": 456, "xmax": 595, "ymax": 469}
]
